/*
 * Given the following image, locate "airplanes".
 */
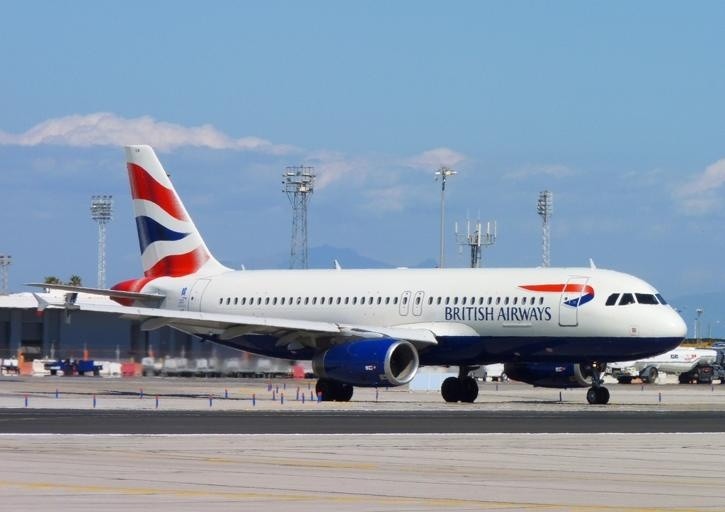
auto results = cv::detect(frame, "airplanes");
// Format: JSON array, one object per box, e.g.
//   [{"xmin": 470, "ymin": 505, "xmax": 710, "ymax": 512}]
[{"xmin": 22, "ymin": 144, "xmax": 687, "ymax": 404}]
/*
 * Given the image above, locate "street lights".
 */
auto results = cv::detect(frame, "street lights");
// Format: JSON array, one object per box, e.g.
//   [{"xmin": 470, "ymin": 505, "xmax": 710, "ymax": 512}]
[
  {"xmin": 90, "ymin": 194, "xmax": 115, "ymax": 288},
  {"xmin": 433, "ymin": 166, "xmax": 458, "ymax": 267},
  {"xmin": 537, "ymin": 189, "xmax": 552, "ymax": 268},
  {"xmin": 0, "ymin": 255, "xmax": 13, "ymax": 294}
]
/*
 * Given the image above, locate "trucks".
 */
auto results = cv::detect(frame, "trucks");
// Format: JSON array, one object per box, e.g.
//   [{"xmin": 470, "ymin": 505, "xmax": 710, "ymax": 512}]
[{"xmin": 606, "ymin": 341, "xmax": 725, "ymax": 384}]
